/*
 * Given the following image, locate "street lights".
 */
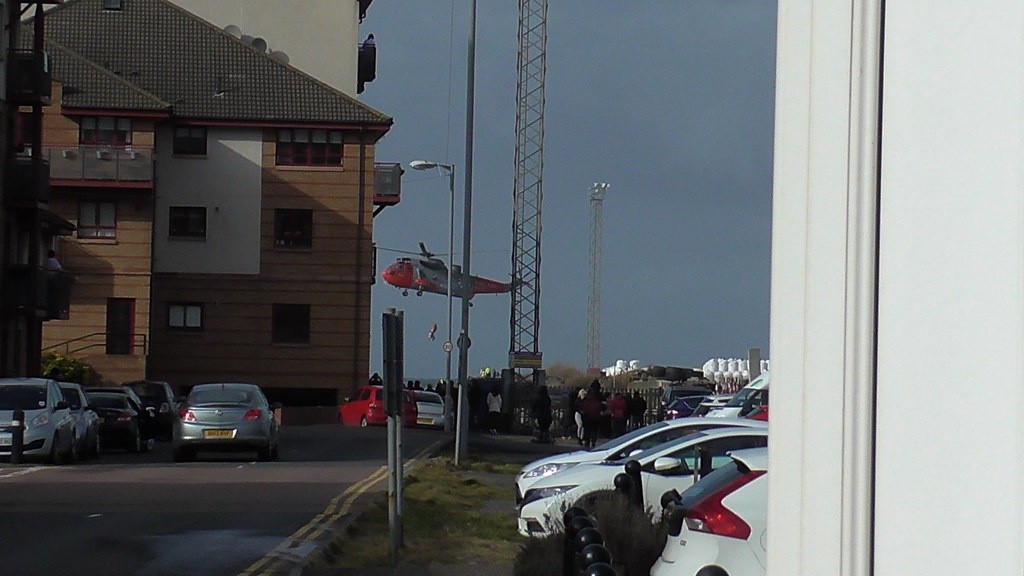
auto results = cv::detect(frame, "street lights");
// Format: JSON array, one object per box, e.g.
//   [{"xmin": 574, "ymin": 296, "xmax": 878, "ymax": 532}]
[{"xmin": 408, "ymin": 160, "xmax": 454, "ymax": 433}]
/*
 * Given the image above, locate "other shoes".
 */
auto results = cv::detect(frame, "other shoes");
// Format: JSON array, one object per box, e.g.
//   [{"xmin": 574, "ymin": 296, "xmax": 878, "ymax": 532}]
[
  {"xmin": 493, "ymin": 429, "xmax": 497, "ymax": 435},
  {"xmin": 579, "ymin": 439, "xmax": 583, "ymax": 446},
  {"xmin": 489, "ymin": 429, "xmax": 493, "ymax": 434},
  {"xmin": 577, "ymin": 437, "xmax": 580, "ymax": 444}
]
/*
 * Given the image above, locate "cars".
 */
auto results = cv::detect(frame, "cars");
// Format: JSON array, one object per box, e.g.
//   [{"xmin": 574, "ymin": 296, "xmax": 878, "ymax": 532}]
[
  {"xmin": 413, "ymin": 390, "xmax": 445, "ymax": 429},
  {"xmin": 513, "ymin": 417, "xmax": 767, "ymax": 505},
  {"xmin": 0, "ymin": 377, "xmax": 77, "ymax": 465},
  {"xmin": 173, "ymin": 383, "xmax": 282, "ymax": 461},
  {"xmin": 337, "ymin": 385, "xmax": 416, "ymax": 428},
  {"xmin": 58, "ymin": 382, "xmax": 101, "ymax": 461},
  {"xmin": 83, "ymin": 385, "xmax": 157, "ymax": 453},
  {"xmin": 515, "ymin": 425, "xmax": 768, "ymax": 540}
]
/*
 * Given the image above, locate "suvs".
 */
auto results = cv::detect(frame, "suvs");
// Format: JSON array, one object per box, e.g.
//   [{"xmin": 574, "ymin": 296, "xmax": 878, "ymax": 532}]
[
  {"xmin": 657, "ymin": 370, "xmax": 769, "ymax": 423},
  {"xmin": 650, "ymin": 446, "xmax": 769, "ymax": 576},
  {"xmin": 120, "ymin": 379, "xmax": 180, "ymax": 439}
]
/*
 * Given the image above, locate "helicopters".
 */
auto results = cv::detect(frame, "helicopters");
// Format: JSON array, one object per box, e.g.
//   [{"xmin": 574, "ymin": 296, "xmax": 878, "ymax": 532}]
[{"xmin": 375, "ymin": 241, "xmax": 522, "ymax": 306}]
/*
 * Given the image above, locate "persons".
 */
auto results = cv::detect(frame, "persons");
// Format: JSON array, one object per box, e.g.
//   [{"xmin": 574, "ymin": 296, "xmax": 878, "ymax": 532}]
[
  {"xmin": 46, "ymin": 250, "xmax": 61, "ymax": 271},
  {"xmin": 370, "ymin": 373, "xmax": 646, "ymax": 449}
]
